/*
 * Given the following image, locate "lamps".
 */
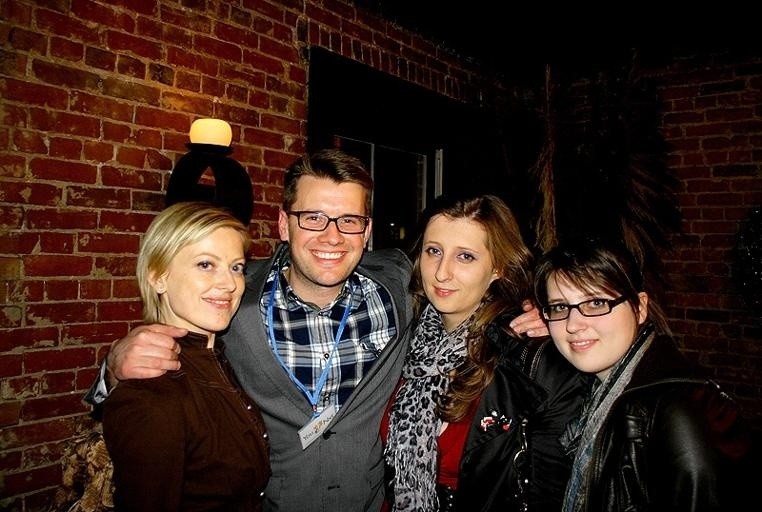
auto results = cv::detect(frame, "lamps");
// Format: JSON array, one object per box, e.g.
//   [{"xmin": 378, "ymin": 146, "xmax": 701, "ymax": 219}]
[{"xmin": 165, "ymin": 118, "xmax": 254, "ymax": 227}]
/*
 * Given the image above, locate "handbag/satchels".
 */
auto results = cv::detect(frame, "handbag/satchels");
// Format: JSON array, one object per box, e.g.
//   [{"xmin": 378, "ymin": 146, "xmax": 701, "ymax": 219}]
[
  {"xmin": 46, "ymin": 432, "xmax": 119, "ymax": 512},
  {"xmin": 500, "ymin": 446, "xmax": 547, "ymax": 511}
]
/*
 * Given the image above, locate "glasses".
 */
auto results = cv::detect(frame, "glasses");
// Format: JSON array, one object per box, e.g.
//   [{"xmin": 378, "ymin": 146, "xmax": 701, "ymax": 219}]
[
  {"xmin": 286, "ymin": 211, "xmax": 369, "ymax": 235},
  {"xmin": 540, "ymin": 294, "xmax": 626, "ymax": 321}
]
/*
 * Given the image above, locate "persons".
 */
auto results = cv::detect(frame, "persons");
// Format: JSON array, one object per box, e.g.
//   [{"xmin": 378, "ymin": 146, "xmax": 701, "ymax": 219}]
[
  {"xmin": 96, "ymin": 194, "xmax": 274, "ymax": 510},
  {"xmin": 529, "ymin": 238, "xmax": 748, "ymax": 510},
  {"xmin": 78, "ymin": 147, "xmax": 553, "ymax": 510},
  {"xmin": 375, "ymin": 185, "xmax": 541, "ymax": 509}
]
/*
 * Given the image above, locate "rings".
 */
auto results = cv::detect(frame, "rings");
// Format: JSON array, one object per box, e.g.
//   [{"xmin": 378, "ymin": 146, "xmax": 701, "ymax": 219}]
[{"xmin": 171, "ymin": 338, "xmax": 178, "ymax": 350}]
